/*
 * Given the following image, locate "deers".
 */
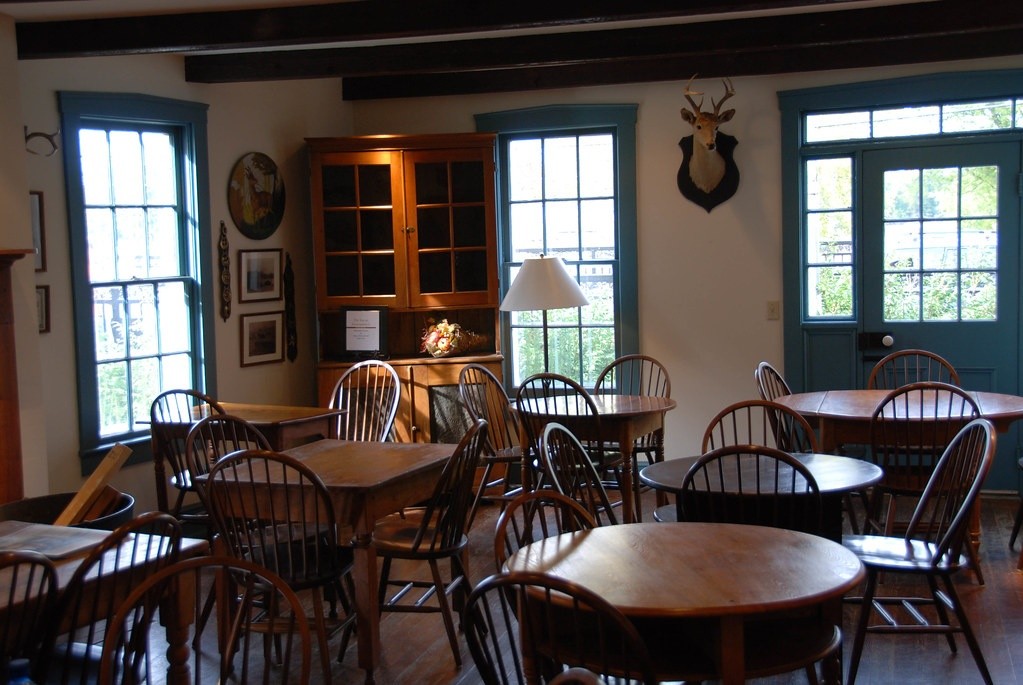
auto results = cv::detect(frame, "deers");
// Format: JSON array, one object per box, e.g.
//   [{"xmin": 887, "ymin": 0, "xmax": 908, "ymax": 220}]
[{"xmin": 679, "ymin": 71, "xmax": 737, "ymax": 194}]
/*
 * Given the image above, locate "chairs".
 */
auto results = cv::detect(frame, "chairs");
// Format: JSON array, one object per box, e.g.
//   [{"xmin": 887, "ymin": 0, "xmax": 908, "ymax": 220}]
[
  {"xmin": 863, "ymin": 381, "xmax": 986, "ymax": 587},
  {"xmin": 0, "ymin": 544, "xmax": 63, "ymax": 685},
  {"xmin": 1008, "ymin": 455, "xmax": 1023, "ymax": 570},
  {"xmin": 839, "ymin": 474, "xmax": 932, "ymax": 684},
  {"xmin": 702, "ymin": 400, "xmax": 821, "ymax": 457},
  {"xmin": 840, "ymin": 416, "xmax": 998, "ymax": 685},
  {"xmin": 679, "ymin": 444, "xmax": 825, "ymax": 537},
  {"xmin": 515, "ymin": 373, "xmax": 623, "ymax": 530},
  {"xmin": 205, "ymin": 450, "xmax": 367, "ymax": 681},
  {"xmin": 440, "ymin": 572, "xmax": 655, "ymax": 685},
  {"xmin": 150, "ymin": 389, "xmax": 226, "ymax": 513},
  {"xmin": 185, "ymin": 413, "xmax": 286, "ymax": 662},
  {"xmin": 753, "ymin": 360, "xmax": 819, "ymax": 454},
  {"xmin": 369, "ymin": 419, "xmax": 488, "ymax": 667},
  {"xmin": 43, "ymin": 512, "xmax": 182, "ymax": 684},
  {"xmin": 95, "ymin": 552, "xmax": 309, "ymax": 685},
  {"xmin": 328, "ymin": 359, "xmax": 404, "ymax": 443},
  {"xmin": 492, "ymin": 489, "xmax": 600, "ymax": 627},
  {"xmin": 538, "ymin": 419, "xmax": 618, "ymax": 527},
  {"xmin": 457, "ymin": 361, "xmax": 562, "ymax": 534},
  {"xmin": 196, "ymin": 391, "xmax": 226, "ymax": 423},
  {"xmin": 866, "ymin": 349, "xmax": 964, "ymax": 391},
  {"xmin": 596, "ymin": 354, "xmax": 669, "ymax": 499}
]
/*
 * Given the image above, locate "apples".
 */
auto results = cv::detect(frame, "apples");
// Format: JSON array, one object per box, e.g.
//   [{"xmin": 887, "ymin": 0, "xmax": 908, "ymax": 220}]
[{"xmin": 428, "ymin": 332, "xmax": 450, "ymax": 351}]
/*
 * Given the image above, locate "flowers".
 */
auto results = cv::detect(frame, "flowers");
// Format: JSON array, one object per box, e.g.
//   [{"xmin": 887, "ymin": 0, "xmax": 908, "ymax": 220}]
[{"xmin": 420, "ymin": 314, "xmax": 472, "ymax": 358}]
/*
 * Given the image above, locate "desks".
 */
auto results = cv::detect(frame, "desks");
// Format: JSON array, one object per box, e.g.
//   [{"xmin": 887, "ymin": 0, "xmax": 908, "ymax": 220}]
[
  {"xmin": 197, "ymin": 438, "xmax": 481, "ymax": 685},
  {"xmin": 501, "ymin": 523, "xmax": 865, "ymax": 684},
  {"xmin": 0, "ymin": 519, "xmax": 210, "ymax": 684},
  {"xmin": 638, "ymin": 449, "xmax": 884, "ymax": 534},
  {"xmin": 508, "ymin": 394, "xmax": 678, "ymax": 523},
  {"xmin": 771, "ymin": 389, "xmax": 1023, "ymax": 624},
  {"xmin": 197, "ymin": 395, "xmax": 349, "ymax": 454}
]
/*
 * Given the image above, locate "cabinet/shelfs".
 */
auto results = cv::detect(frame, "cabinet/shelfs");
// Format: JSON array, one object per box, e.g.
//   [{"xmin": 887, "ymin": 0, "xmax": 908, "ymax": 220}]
[{"xmin": 300, "ymin": 130, "xmax": 506, "ymax": 496}]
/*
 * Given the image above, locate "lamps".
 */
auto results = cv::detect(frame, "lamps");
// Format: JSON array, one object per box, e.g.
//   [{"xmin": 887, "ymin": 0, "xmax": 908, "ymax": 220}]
[{"xmin": 499, "ymin": 251, "xmax": 591, "ymax": 393}]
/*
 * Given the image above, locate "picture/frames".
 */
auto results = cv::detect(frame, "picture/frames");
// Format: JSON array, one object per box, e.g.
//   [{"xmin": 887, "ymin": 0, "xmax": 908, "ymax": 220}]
[
  {"xmin": 34, "ymin": 284, "xmax": 53, "ymax": 337},
  {"xmin": 237, "ymin": 310, "xmax": 289, "ymax": 367},
  {"xmin": 30, "ymin": 191, "xmax": 48, "ymax": 276},
  {"xmin": 236, "ymin": 248, "xmax": 286, "ymax": 304}
]
